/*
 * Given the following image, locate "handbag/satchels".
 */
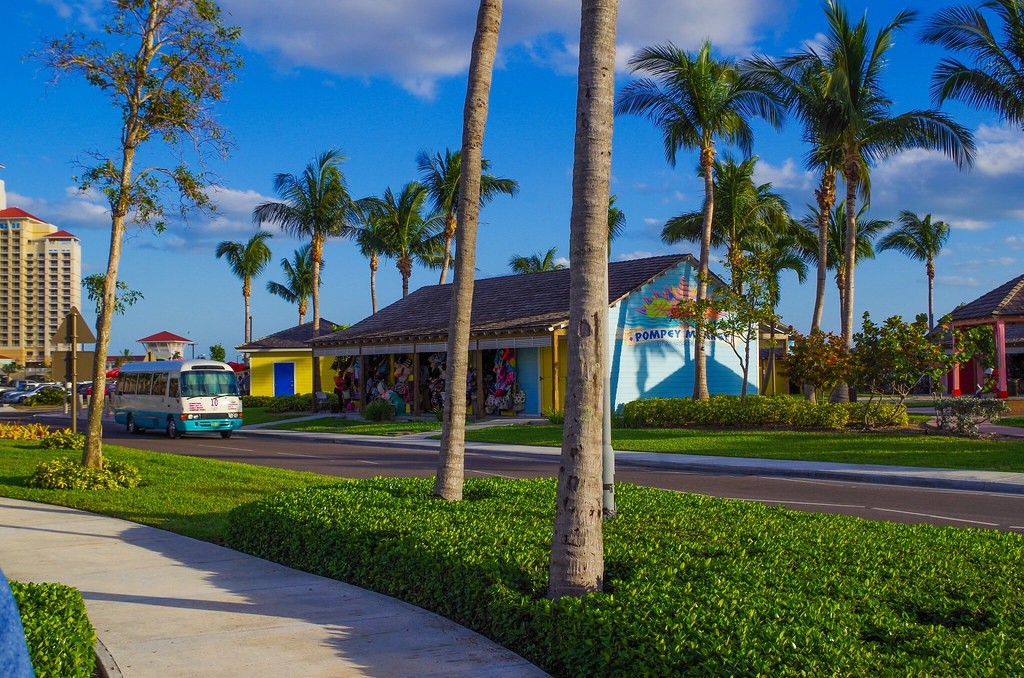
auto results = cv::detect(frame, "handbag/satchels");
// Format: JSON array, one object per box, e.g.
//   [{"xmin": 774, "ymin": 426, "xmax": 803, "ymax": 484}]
[
  {"xmin": 499, "ymin": 391, "xmax": 510, "ymax": 410},
  {"xmin": 485, "ymin": 394, "xmax": 501, "ymax": 408},
  {"xmin": 512, "ymin": 382, "xmax": 527, "ymax": 404}
]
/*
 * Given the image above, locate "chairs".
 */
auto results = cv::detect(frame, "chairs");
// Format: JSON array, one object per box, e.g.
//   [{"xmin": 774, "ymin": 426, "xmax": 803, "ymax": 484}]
[{"xmin": 316, "ymin": 391, "xmax": 330, "ymax": 413}]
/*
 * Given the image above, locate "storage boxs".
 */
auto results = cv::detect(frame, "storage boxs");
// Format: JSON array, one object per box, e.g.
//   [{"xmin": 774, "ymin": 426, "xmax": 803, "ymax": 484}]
[{"xmin": 350, "ymin": 398, "xmax": 361, "ymax": 408}]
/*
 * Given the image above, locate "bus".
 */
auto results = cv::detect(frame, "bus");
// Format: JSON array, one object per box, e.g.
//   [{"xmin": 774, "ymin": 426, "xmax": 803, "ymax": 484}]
[{"xmin": 114, "ymin": 358, "xmax": 245, "ymax": 439}]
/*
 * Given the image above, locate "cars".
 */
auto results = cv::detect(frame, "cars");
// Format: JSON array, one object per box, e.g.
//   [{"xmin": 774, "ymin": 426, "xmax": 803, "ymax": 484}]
[{"xmin": 0, "ymin": 375, "xmax": 128, "ymax": 406}]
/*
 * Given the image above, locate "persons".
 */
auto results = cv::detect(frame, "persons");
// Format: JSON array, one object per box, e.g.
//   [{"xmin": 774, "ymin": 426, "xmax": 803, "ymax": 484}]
[
  {"xmin": 237, "ymin": 371, "xmax": 250, "ymax": 397},
  {"xmin": 334, "ymin": 371, "xmax": 346, "ymax": 414}
]
